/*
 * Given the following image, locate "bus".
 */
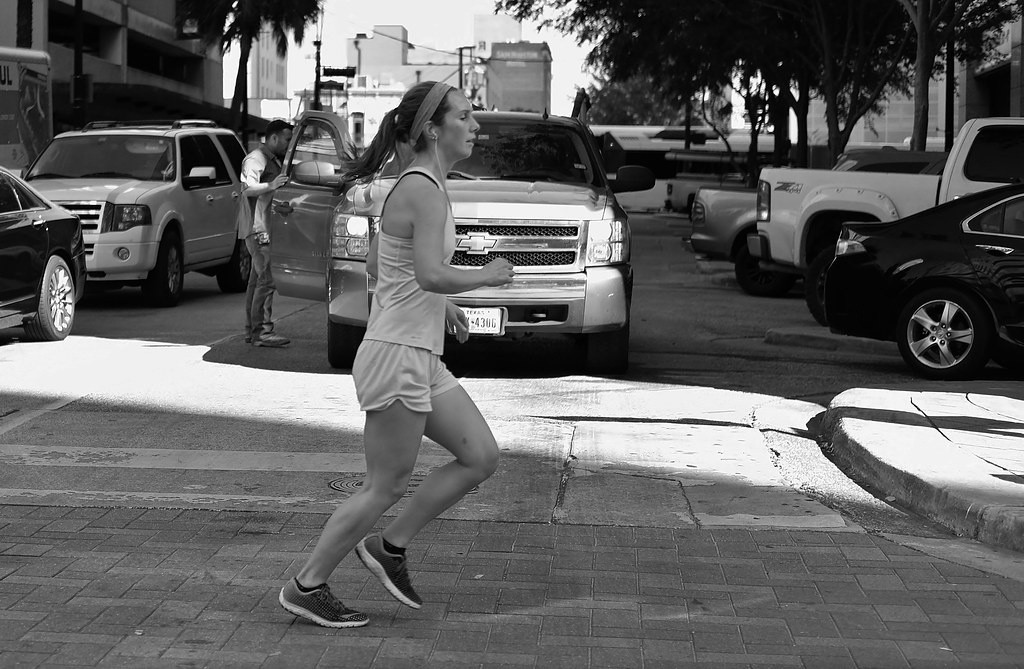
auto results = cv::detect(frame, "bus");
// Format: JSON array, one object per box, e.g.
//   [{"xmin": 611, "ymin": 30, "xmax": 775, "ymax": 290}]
[{"xmin": 588, "ymin": 124, "xmax": 790, "ymax": 216}]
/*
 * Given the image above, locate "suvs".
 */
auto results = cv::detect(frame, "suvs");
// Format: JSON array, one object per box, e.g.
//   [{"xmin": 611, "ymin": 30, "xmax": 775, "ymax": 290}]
[
  {"xmin": 266, "ymin": 106, "xmax": 657, "ymax": 377},
  {"xmin": 13, "ymin": 118, "xmax": 264, "ymax": 307}
]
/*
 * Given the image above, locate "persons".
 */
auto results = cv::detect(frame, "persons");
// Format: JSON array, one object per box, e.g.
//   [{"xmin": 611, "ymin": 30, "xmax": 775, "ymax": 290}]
[
  {"xmin": 239, "ymin": 120, "xmax": 294, "ymax": 347},
  {"xmin": 277, "ymin": 80, "xmax": 517, "ymax": 630}
]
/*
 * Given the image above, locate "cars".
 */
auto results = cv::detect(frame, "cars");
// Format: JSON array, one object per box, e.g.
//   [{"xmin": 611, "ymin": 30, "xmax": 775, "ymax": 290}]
[
  {"xmin": 1, "ymin": 165, "xmax": 90, "ymax": 343},
  {"xmin": 822, "ymin": 182, "xmax": 1024, "ymax": 381}
]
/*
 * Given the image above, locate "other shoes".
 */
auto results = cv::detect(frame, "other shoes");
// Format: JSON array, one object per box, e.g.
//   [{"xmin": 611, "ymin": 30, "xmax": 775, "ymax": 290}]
[
  {"xmin": 253, "ymin": 334, "xmax": 290, "ymax": 347},
  {"xmin": 245, "ymin": 337, "xmax": 252, "ymax": 344}
]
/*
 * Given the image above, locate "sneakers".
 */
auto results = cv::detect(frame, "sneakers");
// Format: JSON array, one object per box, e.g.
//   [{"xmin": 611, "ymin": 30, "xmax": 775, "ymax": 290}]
[
  {"xmin": 279, "ymin": 576, "xmax": 369, "ymax": 629},
  {"xmin": 355, "ymin": 532, "xmax": 423, "ymax": 610}
]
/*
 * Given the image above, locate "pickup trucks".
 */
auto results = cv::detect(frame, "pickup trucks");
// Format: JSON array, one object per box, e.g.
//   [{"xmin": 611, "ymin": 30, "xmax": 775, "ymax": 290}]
[
  {"xmin": 744, "ymin": 115, "xmax": 1024, "ymax": 298},
  {"xmin": 664, "ymin": 137, "xmax": 950, "ymax": 298}
]
